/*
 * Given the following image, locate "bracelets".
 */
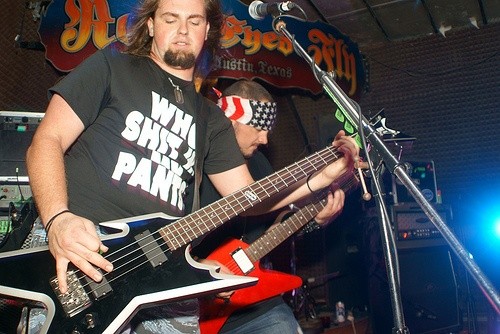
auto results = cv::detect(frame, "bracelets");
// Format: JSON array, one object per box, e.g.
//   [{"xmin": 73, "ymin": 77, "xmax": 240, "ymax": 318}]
[
  {"xmin": 306, "ymin": 173, "xmax": 313, "ymax": 193},
  {"xmin": 44, "ymin": 209, "xmax": 72, "ymax": 243}
]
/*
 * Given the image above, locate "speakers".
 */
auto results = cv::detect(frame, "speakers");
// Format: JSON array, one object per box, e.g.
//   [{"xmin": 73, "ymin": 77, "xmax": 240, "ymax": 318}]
[{"xmin": 365, "ymin": 246, "xmax": 463, "ymax": 334}]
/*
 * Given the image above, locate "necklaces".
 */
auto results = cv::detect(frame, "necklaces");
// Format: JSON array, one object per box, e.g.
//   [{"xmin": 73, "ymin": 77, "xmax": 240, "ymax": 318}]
[{"xmin": 160, "ymin": 68, "xmax": 193, "ymax": 105}]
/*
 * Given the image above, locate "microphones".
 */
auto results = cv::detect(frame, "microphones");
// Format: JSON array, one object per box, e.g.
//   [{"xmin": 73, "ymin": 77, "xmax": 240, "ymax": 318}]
[{"xmin": 248, "ymin": 0, "xmax": 296, "ymax": 20}]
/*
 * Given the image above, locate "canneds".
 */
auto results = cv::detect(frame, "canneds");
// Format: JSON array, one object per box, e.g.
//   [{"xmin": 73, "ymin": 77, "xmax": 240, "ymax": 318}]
[{"xmin": 335, "ymin": 301, "xmax": 346, "ymax": 322}]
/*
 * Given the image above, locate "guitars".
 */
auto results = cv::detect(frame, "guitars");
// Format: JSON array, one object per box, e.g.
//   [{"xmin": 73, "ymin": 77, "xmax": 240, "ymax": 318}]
[{"xmin": 0, "ymin": 108, "xmax": 400, "ymax": 334}]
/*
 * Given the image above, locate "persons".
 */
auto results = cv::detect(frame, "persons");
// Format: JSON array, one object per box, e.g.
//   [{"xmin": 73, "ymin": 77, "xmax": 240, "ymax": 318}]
[
  {"xmin": 188, "ymin": 82, "xmax": 346, "ymax": 334},
  {"xmin": 25, "ymin": 0, "xmax": 359, "ymax": 334}
]
